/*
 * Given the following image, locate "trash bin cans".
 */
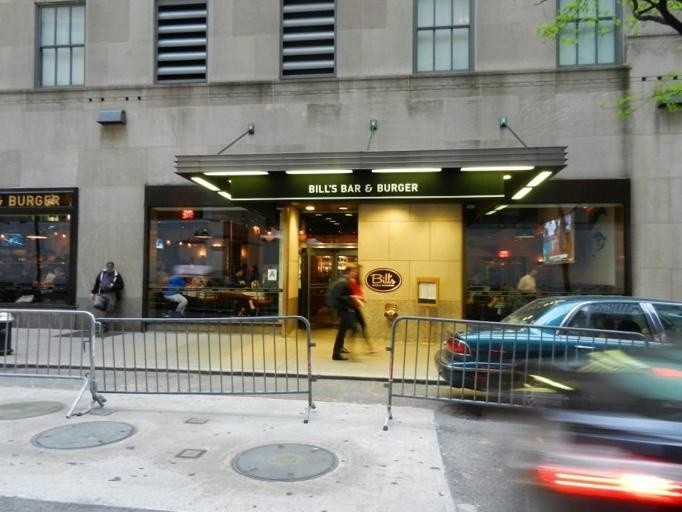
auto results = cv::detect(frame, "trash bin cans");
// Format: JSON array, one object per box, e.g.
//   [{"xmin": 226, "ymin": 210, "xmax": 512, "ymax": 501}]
[{"xmin": 0, "ymin": 311, "xmax": 15, "ymax": 356}]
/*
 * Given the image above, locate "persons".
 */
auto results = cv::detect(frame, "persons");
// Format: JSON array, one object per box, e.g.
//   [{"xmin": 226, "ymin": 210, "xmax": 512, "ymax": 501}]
[
  {"xmin": 343, "ymin": 266, "xmax": 366, "ymax": 338},
  {"xmin": 248, "ymin": 278, "xmax": 263, "ymax": 311},
  {"xmin": 91, "ymin": 261, "xmax": 124, "ymax": 335},
  {"xmin": 162, "ymin": 271, "xmax": 189, "ymax": 315},
  {"xmin": 326, "ymin": 265, "xmax": 372, "ymax": 362},
  {"xmin": 513, "ymin": 266, "xmax": 538, "ymax": 299},
  {"xmin": 551, "ymin": 232, "xmax": 561, "ymax": 257},
  {"xmin": 22, "ymin": 248, "xmax": 69, "ymax": 292},
  {"xmin": 228, "ymin": 267, "xmax": 245, "ymax": 316}
]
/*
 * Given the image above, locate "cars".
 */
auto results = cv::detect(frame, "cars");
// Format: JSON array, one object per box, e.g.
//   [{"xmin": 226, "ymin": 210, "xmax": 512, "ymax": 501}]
[{"xmin": 434, "ymin": 293, "xmax": 682, "ymax": 391}]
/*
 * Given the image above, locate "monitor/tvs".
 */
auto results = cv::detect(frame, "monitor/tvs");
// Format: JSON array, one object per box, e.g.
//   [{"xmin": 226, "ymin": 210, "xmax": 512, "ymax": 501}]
[
  {"xmin": 0, "ymin": 232, "xmax": 29, "ymax": 250},
  {"xmin": 542, "ymin": 212, "xmax": 576, "ymax": 264},
  {"xmin": 156, "ymin": 238, "xmax": 164, "ymax": 250}
]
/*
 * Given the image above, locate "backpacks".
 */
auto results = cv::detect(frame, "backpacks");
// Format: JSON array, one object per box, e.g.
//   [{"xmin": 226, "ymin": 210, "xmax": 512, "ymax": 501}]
[{"xmin": 326, "ymin": 280, "xmax": 337, "ymax": 308}]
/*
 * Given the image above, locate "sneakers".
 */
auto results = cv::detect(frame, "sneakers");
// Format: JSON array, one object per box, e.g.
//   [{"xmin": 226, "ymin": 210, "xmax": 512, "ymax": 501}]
[
  {"xmin": 341, "ymin": 347, "xmax": 350, "ymax": 353},
  {"xmin": 97, "ymin": 327, "xmax": 108, "ymax": 333},
  {"xmin": 332, "ymin": 355, "xmax": 349, "ymax": 360}
]
